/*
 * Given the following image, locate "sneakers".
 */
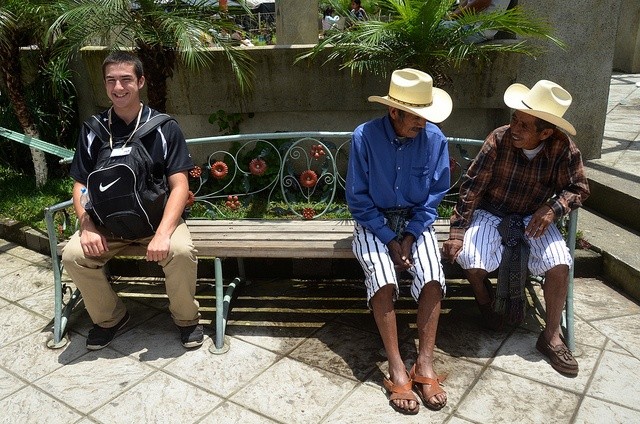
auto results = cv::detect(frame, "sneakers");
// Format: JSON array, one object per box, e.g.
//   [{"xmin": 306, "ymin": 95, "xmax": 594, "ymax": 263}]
[
  {"xmin": 177, "ymin": 326, "xmax": 203, "ymax": 348},
  {"xmin": 86, "ymin": 312, "xmax": 130, "ymax": 349}
]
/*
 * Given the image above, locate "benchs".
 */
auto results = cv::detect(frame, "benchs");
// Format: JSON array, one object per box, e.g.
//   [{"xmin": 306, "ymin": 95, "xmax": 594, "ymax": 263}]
[{"xmin": 44, "ymin": 132, "xmax": 583, "ymax": 358}]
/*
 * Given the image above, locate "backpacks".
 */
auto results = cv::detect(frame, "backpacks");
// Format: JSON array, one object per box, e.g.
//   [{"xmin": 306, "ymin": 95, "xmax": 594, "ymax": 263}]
[{"xmin": 83, "ymin": 114, "xmax": 179, "ymax": 240}]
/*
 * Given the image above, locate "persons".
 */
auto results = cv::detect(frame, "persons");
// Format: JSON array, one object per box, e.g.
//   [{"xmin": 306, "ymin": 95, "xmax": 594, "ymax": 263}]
[
  {"xmin": 62, "ymin": 51, "xmax": 204, "ymax": 350},
  {"xmin": 442, "ymin": 79, "xmax": 590, "ymax": 377},
  {"xmin": 350, "ymin": 1, "xmax": 368, "ymax": 24},
  {"xmin": 210, "ymin": 1, "xmax": 234, "ymax": 22},
  {"xmin": 321, "ymin": 6, "xmax": 340, "ymax": 33},
  {"xmin": 345, "ymin": 68, "xmax": 453, "ymax": 415}
]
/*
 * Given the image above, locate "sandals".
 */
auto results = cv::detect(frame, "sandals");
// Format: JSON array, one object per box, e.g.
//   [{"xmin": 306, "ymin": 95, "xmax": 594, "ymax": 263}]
[
  {"xmin": 382, "ymin": 370, "xmax": 420, "ymax": 414},
  {"xmin": 410, "ymin": 364, "xmax": 447, "ymax": 410}
]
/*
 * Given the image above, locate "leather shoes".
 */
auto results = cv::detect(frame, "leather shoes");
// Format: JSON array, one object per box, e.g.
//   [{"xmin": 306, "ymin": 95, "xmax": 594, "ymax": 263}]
[
  {"xmin": 477, "ymin": 281, "xmax": 496, "ymax": 329},
  {"xmin": 536, "ymin": 331, "xmax": 578, "ymax": 374}
]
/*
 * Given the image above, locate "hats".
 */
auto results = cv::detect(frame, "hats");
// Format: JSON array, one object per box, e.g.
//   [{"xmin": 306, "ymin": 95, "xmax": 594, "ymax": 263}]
[
  {"xmin": 504, "ymin": 80, "xmax": 576, "ymax": 137},
  {"xmin": 368, "ymin": 68, "xmax": 453, "ymax": 124}
]
